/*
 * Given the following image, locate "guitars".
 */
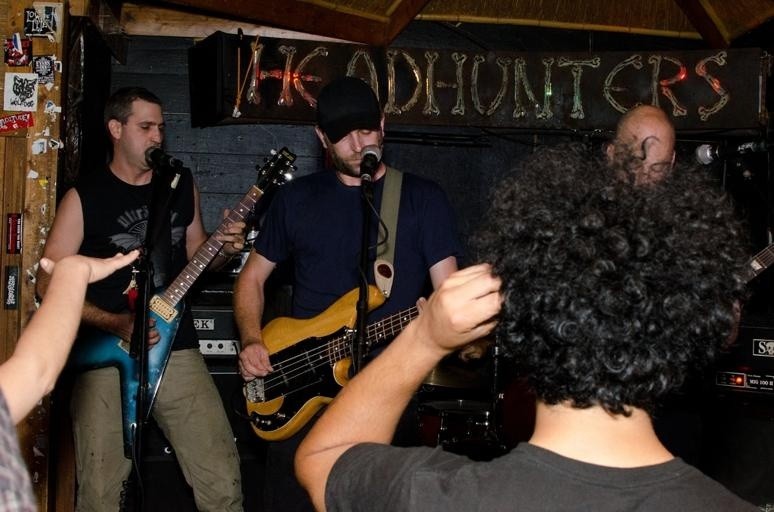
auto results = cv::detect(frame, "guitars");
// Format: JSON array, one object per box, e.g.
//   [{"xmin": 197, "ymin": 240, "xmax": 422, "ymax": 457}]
[
  {"xmin": 500, "ymin": 242, "xmax": 774, "ymax": 444},
  {"xmin": 244, "ymin": 286, "xmax": 428, "ymax": 441},
  {"xmin": 70, "ymin": 146, "xmax": 299, "ymax": 462}
]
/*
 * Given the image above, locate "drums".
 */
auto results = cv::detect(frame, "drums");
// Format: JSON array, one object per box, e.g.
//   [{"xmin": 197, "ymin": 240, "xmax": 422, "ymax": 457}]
[{"xmin": 420, "ymin": 400, "xmax": 496, "ymax": 460}]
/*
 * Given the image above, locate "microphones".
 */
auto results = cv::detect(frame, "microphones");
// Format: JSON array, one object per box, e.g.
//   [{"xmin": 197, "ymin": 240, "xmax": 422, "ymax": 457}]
[
  {"xmin": 144, "ymin": 147, "xmax": 184, "ymax": 173},
  {"xmin": 358, "ymin": 146, "xmax": 383, "ymax": 186},
  {"xmin": 695, "ymin": 142, "xmax": 764, "ymax": 166}
]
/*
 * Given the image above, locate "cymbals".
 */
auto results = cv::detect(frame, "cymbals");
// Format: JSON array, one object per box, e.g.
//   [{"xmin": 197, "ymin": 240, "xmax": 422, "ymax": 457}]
[{"xmin": 421, "ymin": 354, "xmax": 494, "ymax": 387}]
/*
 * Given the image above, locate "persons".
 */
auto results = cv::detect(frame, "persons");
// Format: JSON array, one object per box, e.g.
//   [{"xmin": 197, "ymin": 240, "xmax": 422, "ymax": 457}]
[
  {"xmin": 292, "ymin": 133, "xmax": 771, "ymax": 511},
  {"xmin": 33, "ymin": 87, "xmax": 246, "ymax": 509},
  {"xmin": 602, "ymin": 105, "xmax": 677, "ymax": 185},
  {"xmin": 0, "ymin": 248, "xmax": 143, "ymax": 512},
  {"xmin": 231, "ymin": 75, "xmax": 489, "ymax": 453}
]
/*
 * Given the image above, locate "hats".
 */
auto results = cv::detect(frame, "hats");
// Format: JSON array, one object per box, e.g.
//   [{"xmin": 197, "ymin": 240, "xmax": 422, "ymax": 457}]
[{"xmin": 313, "ymin": 75, "xmax": 384, "ymax": 146}]
